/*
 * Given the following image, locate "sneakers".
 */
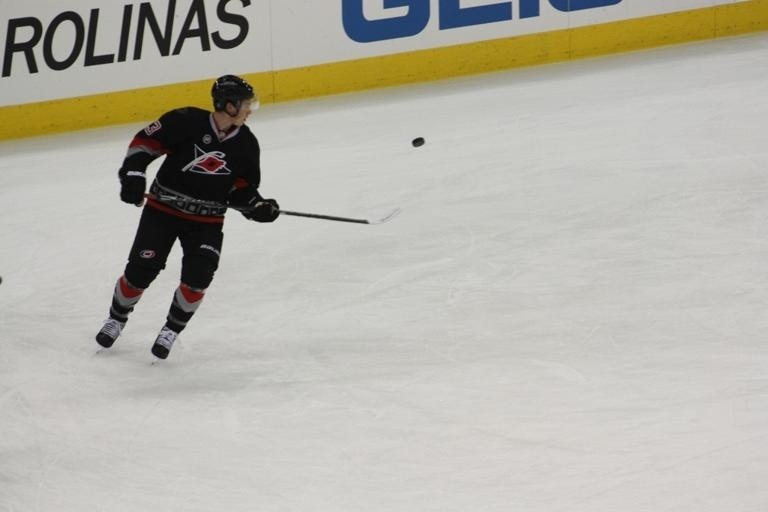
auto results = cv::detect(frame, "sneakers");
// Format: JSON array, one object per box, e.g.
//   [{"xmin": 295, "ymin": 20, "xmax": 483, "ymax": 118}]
[
  {"xmin": 94, "ymin": 314, "xmax": 126, "ymax": 349},
  {"xmin": 149, "ymin": 325, "xmax": 179, "ymax": 361}
]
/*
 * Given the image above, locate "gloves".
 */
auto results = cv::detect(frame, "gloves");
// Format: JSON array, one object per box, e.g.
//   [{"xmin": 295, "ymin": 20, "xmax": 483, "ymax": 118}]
[
  {"xmin": 229, "ymin": 183, "xmax": 282, "ymax": 226},
  {"xmin": 116, "ymin": 152, "xmax": 155, "ymax": 209}
]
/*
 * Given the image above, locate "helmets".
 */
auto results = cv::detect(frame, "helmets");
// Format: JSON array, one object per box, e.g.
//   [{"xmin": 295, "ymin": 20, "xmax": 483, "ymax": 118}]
[{"xmin": 211, "ymin": 73, "xmax": 257, "ymax": 101}]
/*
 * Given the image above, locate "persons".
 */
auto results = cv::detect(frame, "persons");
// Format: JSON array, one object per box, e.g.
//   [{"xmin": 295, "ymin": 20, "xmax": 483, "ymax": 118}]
[{"xmin": 95, "ymin": 74, "xmax": 282, "ymax": 361}]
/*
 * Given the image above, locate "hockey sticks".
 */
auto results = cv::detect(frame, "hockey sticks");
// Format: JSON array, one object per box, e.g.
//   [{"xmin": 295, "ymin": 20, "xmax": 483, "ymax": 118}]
[{"xmin": 143, "ymin": 194, "xmax": 408, "ymax": 225}]
